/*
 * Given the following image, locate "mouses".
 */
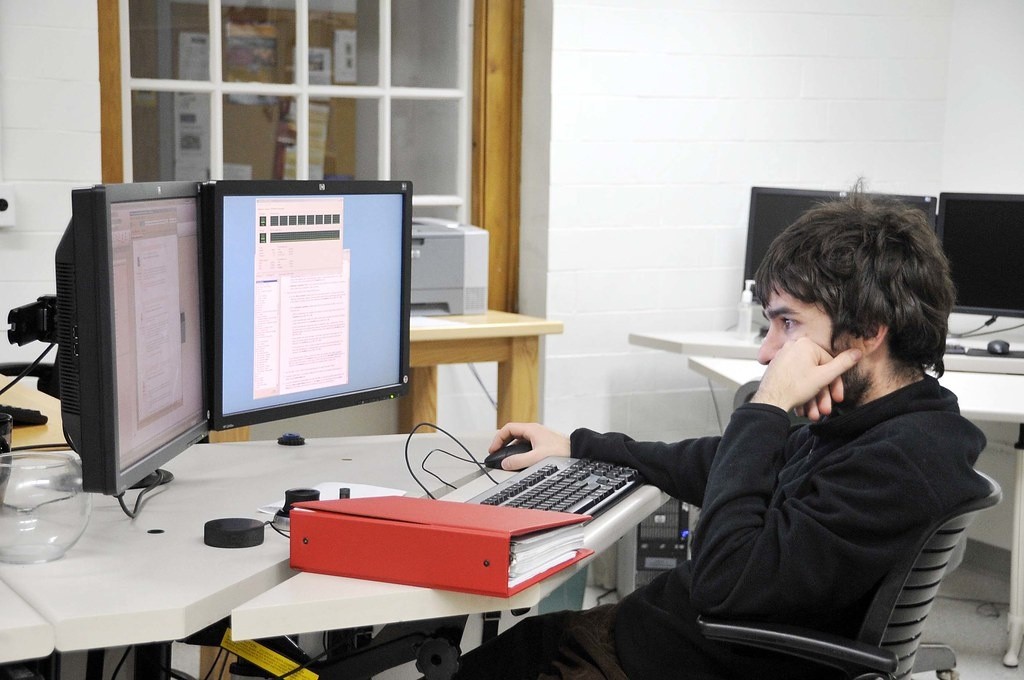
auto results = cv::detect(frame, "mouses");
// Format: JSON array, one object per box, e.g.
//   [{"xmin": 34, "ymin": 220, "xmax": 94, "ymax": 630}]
[
  {"xmin": 988, "ymin": 340, "xmax": 1009, "ymax": 354},
  {"xmin": 484, "ymin": 444, "xmax": 532, "ymax": 472}
]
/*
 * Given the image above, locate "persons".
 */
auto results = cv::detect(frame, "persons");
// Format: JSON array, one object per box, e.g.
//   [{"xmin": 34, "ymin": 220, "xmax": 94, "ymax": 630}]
[{"xmin": 452, "ymin": 179, "xmax": 989, "ymax": 680}]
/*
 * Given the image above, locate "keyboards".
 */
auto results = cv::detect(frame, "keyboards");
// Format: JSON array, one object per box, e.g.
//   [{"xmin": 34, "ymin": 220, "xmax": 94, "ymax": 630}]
[
  {"xmin": 464, "ymin": 455, "xmax": 645, "ymax": 527},
  {"xmin": 944, "ymin": 345, "xmax": 966, "ymax": 354}
]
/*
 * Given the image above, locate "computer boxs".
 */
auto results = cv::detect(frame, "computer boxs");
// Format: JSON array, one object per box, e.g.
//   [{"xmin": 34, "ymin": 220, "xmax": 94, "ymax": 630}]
[{"xmin": 616, "ymin": 497, "xmax": 689, "ymax": 601}]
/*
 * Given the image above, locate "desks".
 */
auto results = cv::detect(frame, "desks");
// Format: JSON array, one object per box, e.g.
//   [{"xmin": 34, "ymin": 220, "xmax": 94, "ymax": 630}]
[
  {"xmin": 0, "ymin": 309, "xmax": 564, "ymax": 680},
  {"xmin": 630, "ymin": 324, "xmax": 1024, "ymax": 668},
  {"xmin": 0, "ymin": 433, "xmax": 671, "ymax": 680}
]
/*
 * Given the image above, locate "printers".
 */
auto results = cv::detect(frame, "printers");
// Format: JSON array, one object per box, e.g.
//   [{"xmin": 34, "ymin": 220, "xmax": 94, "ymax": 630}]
[{"xmin": 411, "ymin": 216, "xmax": 490, "ymax": 316}]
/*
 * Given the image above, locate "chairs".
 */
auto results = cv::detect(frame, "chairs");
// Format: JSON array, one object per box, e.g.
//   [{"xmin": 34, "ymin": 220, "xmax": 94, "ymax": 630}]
[{"xmin": 697, "ymin": 468, "xmax": 1003, "ymax": 680}]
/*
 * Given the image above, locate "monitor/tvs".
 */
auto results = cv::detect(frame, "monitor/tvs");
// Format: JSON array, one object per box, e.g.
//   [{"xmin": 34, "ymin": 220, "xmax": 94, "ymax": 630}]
[
  {"xmin": 742, "ymin": 187, "xmax": 937, "ymax": 301},
  {"xmin": 55, "ymin": 181, "xmax": 210, "ymax": 496},
  {"xmin": 209, "ymin": 180, "xmax": 413, "ymax": 431},
  {"xmin": 937, "ymin": 193, "xmax": 1024, "ymax": 318}
]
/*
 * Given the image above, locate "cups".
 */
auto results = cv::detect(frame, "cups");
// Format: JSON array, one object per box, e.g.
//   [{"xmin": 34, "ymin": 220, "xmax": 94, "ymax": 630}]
[{"xmin": 0, "ymin": 452, "xmax": 92, "ymax": 565}]
